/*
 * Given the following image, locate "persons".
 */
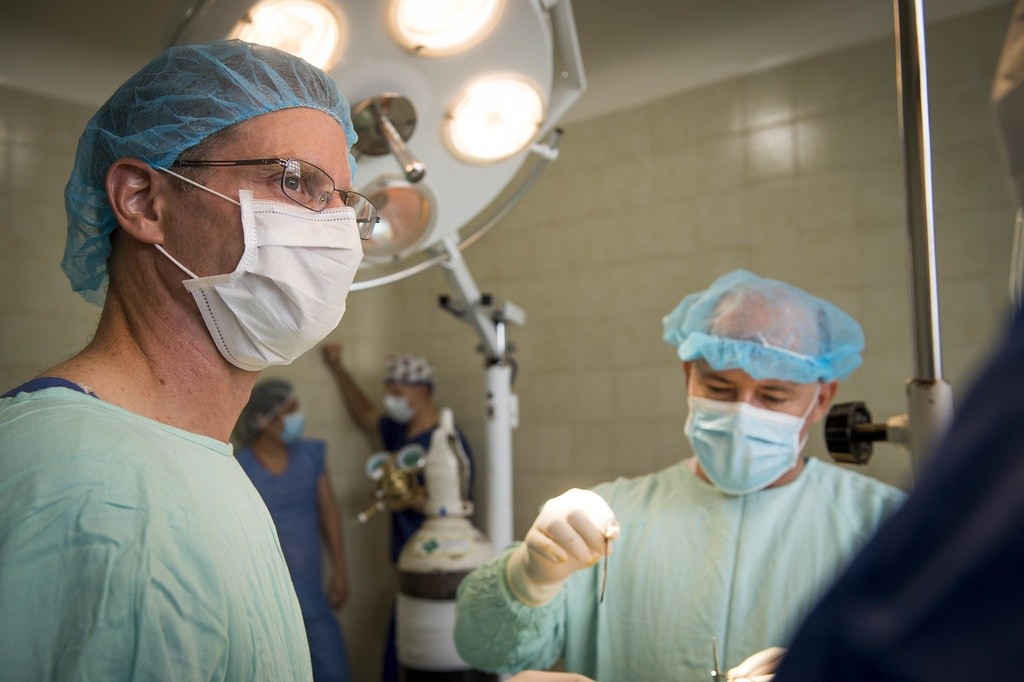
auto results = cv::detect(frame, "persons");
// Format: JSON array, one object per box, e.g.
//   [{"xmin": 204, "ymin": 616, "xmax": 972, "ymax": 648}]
[
  {"xmin": 454, "ymin": 269, "xmax": 911, "ymax": 682},
  {"xmin": 233, "ymin": 378, "xmax": 352, "ymax": 682},
  {"xmin": 322, "ymin": 343, "xmax": 475, "ymax": 682},
  {"xmin": 0, "ymin": 39, "xmax": 383, "ymax": 682}
]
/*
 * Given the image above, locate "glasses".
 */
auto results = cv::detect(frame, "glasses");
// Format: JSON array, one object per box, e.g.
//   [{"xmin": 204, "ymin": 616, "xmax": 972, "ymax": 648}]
[{"xmin": 176, "ymin": 156, "xmax": 381, "ymax": 241}]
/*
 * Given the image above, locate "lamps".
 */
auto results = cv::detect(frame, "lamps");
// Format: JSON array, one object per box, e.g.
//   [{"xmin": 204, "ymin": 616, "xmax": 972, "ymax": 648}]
[{"xmin": 165, "ymin": 0, "xmax": 589, "ymax": 293}]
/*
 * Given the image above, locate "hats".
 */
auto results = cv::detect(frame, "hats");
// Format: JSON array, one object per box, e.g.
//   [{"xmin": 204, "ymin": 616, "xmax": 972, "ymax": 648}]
[
  {"xmin": 238, "ymin": 377, "xmax": 295, "ymax": 435},
  {"xmin": 383, "ymin": 351, "xmax": 437, "ymax": 388}
]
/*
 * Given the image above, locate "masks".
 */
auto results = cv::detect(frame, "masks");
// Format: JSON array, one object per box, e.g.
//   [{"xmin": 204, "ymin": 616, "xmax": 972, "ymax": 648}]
[
  {"xmin": 268, "ymin": 410, "xmax": 307, "ymax": 445},
  {"xmin": 685, "ymin": 368, "xmax": 822, "ymax": 494},
  {"xmin": 384, "ymin": 390, "xmax": 416, "ymax": 424},
  {"xmin": 144, "ymin": 165, "xmax": 364, "ymax": 372}
]
